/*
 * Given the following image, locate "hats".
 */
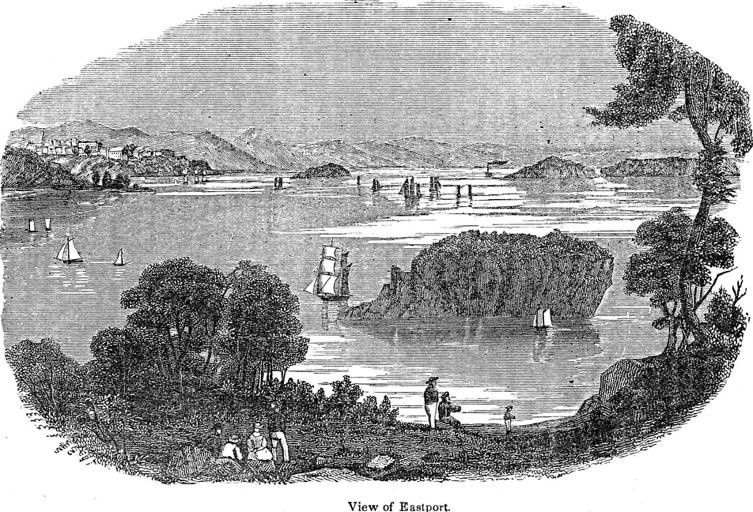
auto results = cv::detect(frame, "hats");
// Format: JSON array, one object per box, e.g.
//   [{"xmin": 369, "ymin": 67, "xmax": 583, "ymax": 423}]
[
  {"xmin": 229, "ymin": 436, "xmax": 240, "ymax": 443},
  {"xmin": 425, "ymin": 377, "xmax": 438, "ymax": 384},
  {"xmin": 252, "ymin": 423, "xmax": 263, "ymax": 429}
]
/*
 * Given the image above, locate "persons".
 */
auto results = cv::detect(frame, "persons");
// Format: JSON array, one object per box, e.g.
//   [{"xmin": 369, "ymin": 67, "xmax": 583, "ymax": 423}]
[
  {"xmin": 505, "ymin": 404, "xmax": 516, "ymax": 434},
  {"xmin": 207, "ymin": 400, "xmax": 289, "ymax": 462},
  {"xmin": 439, "ymin": 391, "xmax": 463, "ymax": 430},
  {"xmin": 425, "ymin": 376, "xmax": 439, "ymax": 431}
]
